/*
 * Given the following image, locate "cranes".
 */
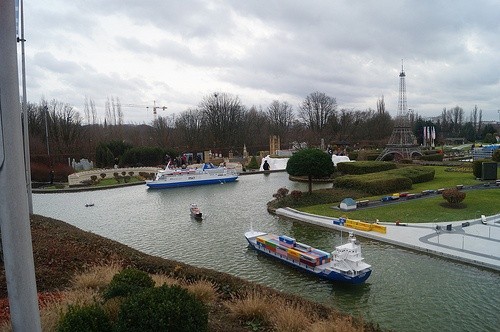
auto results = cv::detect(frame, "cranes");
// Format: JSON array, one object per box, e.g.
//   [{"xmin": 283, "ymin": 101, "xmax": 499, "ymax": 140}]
[{"xmin": 146, "ymin": 100, "xmax": 168, "ymax": 120}]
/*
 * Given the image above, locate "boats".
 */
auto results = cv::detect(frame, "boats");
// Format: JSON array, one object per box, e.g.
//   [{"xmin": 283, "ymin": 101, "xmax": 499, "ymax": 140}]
[
  {"xmin": 144, "ymin": 160, "xmax": 239, "ymax": 190},
  {"xmin": 244, "ymin": 227, "xmax": 373, "ymax": 285},
  {"xmin": 189, "ymin": 203, "xmax": 203, "ymax": 219}
]
